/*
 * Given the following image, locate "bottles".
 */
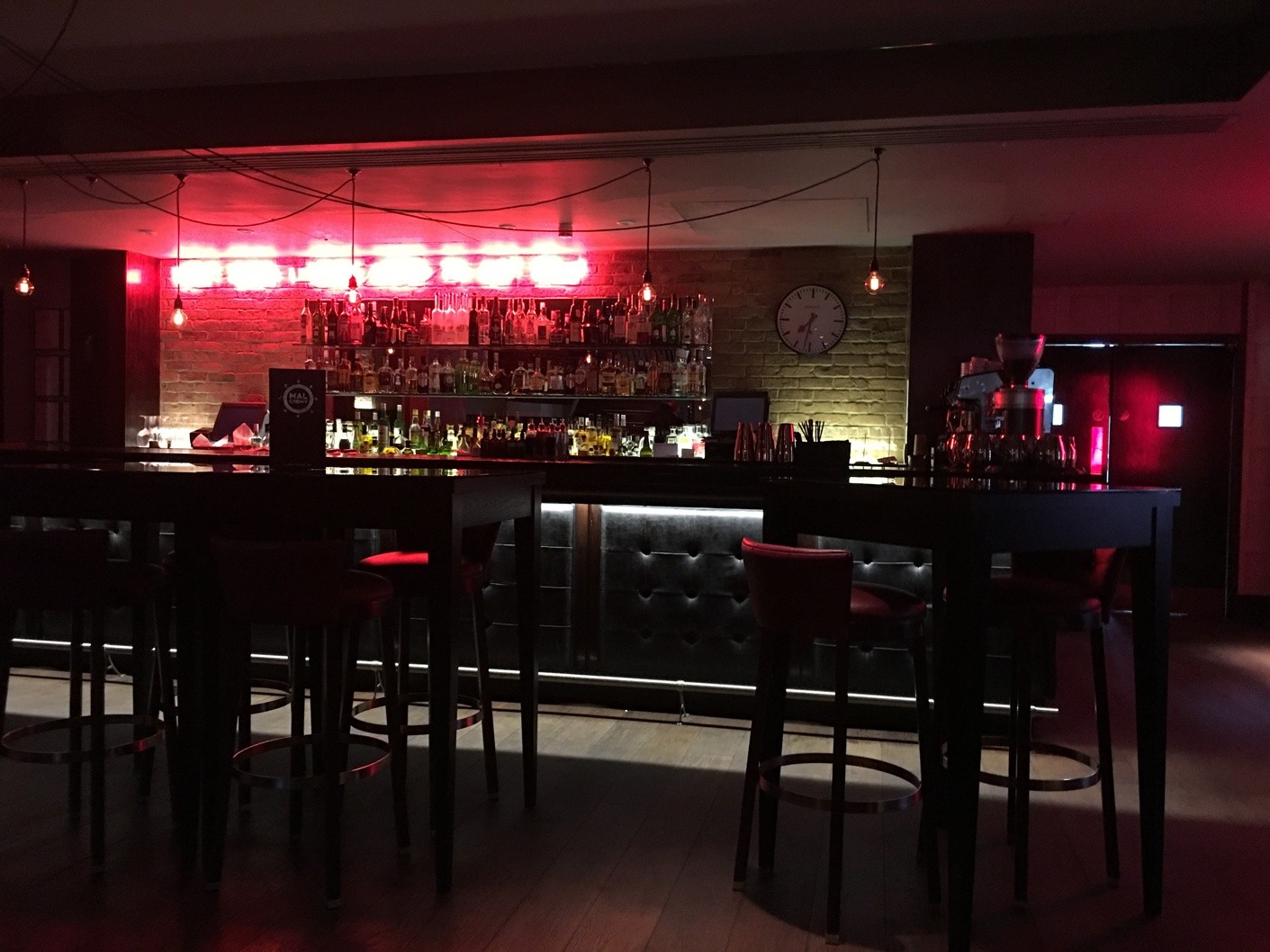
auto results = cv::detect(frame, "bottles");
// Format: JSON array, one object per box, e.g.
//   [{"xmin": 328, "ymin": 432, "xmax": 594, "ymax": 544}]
[
  {"xmin": 305, "ymin": 350, "xmax": 707, "ymax": 398},
  {"xmin": 327, "ymin": 403, "xmax": 711, "ymax": 457},
  {"xmin": 300, "ymin": 292, "xmax": 713, "ymax": 346},
  {"xmin": 251, "ymin": 423, "xmax": 262, "ymax": 449},
  {"xmin": 907, "ymin": 434, "xmax": 1077, "ymax": 473}
]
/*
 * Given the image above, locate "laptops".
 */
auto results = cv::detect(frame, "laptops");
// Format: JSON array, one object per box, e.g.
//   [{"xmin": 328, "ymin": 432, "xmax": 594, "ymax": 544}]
[
  {"xmin": 711, "ymin": 391, "xmax": 769, "ymax": 437},
  {"xmin": 211, "ymin": 402, "xmax": 267, "ymax": 441}
]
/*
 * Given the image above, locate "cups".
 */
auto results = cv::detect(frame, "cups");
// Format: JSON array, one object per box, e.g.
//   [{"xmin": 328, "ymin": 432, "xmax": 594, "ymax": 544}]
[
  {"xmin": 735, "ymin": 421, "xmax": 795, "ymax": 463},
  {"xmin": 960, "ymin": 357, "xmax": 1001, "ymax": 377}
]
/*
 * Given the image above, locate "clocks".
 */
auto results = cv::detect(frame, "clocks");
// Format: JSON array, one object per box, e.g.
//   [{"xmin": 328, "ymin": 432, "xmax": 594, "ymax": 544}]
[{"xmin": 775, "ymin": 284, "xmax": 847, "ymax": 355}]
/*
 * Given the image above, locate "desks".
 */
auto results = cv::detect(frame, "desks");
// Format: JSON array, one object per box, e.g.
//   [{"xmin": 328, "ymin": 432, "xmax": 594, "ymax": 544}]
[
  {"xmin": 746, "ymin": 473, "xmax": 1183, "ymax": 919},
  {"xmin": 0, "ymin": 459, "xmax": 548, "ymax": 896}
]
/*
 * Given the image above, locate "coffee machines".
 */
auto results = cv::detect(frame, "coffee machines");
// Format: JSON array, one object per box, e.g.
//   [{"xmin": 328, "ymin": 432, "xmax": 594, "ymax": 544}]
[{"xmin": 992, "ymin": 332, "xmax": 1046, "ymax": 448}]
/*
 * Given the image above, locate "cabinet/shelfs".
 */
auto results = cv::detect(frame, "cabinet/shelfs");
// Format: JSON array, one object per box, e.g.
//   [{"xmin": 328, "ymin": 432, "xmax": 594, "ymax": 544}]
[{"xmin": 293, "ymin": 343, "xmax": 714, "ymax": 402}]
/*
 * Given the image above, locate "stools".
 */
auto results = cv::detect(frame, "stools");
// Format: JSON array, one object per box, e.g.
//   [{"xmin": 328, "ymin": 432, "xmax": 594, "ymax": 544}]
[
  {"xmin": 0, "ymin": 527, "xmax": 167, "ymax": 875},
  {"xmin": 979, "ymin": 545, "xmax": 1125, "ymax": 899},
  {"xmin": 206, "ymin": 532, "xmax": 411, "ymax": 911},
  {"xmin": 349, "ymin": 518, "xmax": 501, "ymax": 861},
  {"xmin": 738, "ymin": 538, "xmax": 941, "ymax": 937}
]
